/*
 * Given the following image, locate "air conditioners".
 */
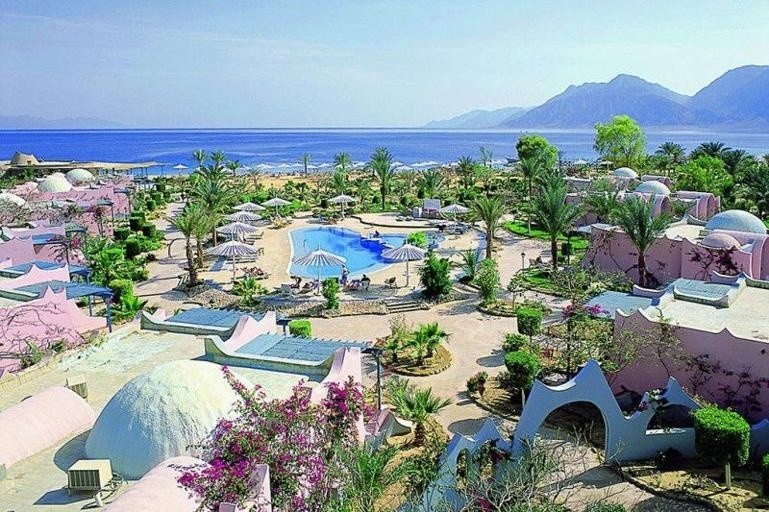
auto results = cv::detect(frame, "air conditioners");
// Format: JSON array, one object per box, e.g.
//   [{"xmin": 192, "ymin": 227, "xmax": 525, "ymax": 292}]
[{"xmin": 68, "ymin": 460, "xmax": 112, "ymax": 490}]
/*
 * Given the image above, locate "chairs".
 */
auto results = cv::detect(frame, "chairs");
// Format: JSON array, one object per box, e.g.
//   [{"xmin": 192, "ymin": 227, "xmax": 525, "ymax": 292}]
[{"xmin": 240, "ymin": 268, "xmax": 397, "ymax": 290}]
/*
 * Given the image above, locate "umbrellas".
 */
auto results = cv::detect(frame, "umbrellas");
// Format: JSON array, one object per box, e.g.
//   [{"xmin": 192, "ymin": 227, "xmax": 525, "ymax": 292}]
[
  {"xmin": 224, "ymin": 209, "xmax": 262, "ymax": 239},
  {"xmin": 599, "ymin": 160, "xmax": 614, "ymax": 164},
  {"xmin": 439, "ymin": 200, "xmax": 469, "ymax": 229},
  {"xmin": 174, "ymin": 163, "xmax": 188, "ymax": 169},
  {"xmin": 293, "ymin": 244, "xmax": 347, "ymax": 294},
  {"xmin": 328, "ymin": 191, "xmax": 355, "ymax": 212},
  {"xmin": 215, "ymin": 218, "xmax": 262, "ymax": 240},
  {"xmin": 575, "ymin": 159, "xmax": 588, "ymax": 164},
  {"xmin": 231, "ymin": 200, "xmax": 266, "ymax": 212},
  {"xmin": 260, "ymin": 195, "xmax": 292, "ymax": 214},
  {"xmin": 204, "ymin": 236, "xmax": 257, "ymax": 280},
  {"xmin": 380, "ymin": 239, "xmax": 426, "ymax": 286}
]
